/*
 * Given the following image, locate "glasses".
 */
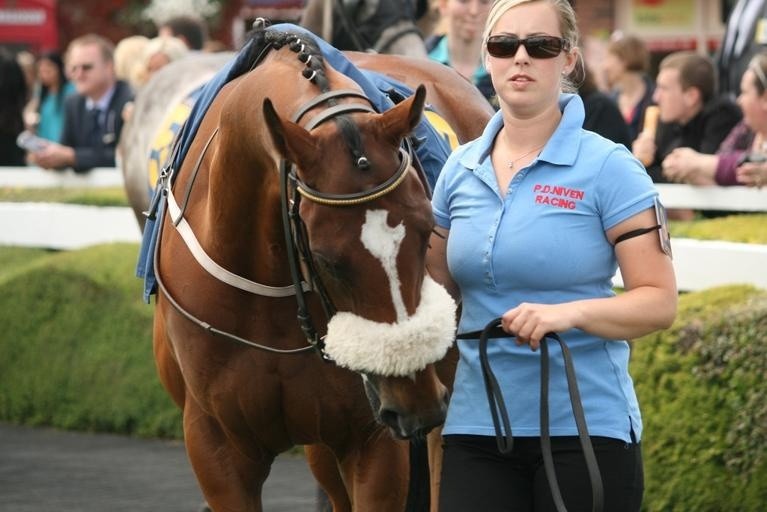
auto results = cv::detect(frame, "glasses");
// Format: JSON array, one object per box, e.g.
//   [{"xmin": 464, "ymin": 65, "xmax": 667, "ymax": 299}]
[
  {"xmin": 484, "ymin": 31, "xmax": 571, "ymax": 60},
  {"xmin": 65, "ymin": 59, "xmax": 103, "ymax": 75}
]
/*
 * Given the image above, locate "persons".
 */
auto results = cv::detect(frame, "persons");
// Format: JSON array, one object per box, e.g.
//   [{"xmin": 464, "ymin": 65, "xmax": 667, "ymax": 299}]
[
  {"xmin": 420, "ymin": 0, "xmax": 678, "ymax": 511},
  {"xmin": 1, "ymin": 2, "xmax": 767, "ymax": 186}
]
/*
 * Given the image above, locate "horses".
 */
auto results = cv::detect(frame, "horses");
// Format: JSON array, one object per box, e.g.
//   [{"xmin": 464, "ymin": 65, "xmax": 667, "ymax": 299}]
[
  {"xmin": 148, "ymin": 23, "xmax": 499, "ymax": 512},
  {"xmin": 117, "ymin": 0, "xmax": 432, "ymax": 239}
]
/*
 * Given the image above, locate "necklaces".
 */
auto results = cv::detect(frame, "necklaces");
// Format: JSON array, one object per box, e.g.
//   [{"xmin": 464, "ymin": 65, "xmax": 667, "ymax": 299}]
[{"xmin": 501, "ymin": 143, "xmax": 545, "ymax": 169}]
